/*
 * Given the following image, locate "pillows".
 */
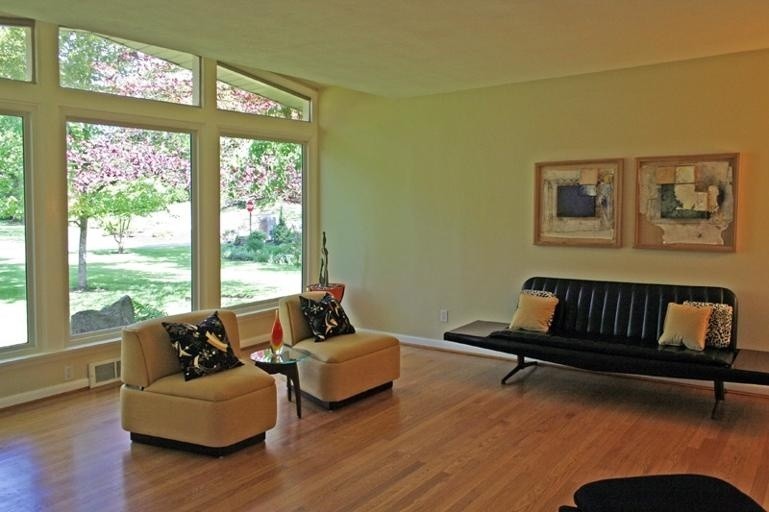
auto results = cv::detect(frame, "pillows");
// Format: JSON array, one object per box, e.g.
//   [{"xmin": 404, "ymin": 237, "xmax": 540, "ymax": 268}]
[
  {"xmin": 160, "ymin": 311, "xmax": 246, "ymax": 382},
  {"xmin": 520, "ymin": 290, "xmax": 556, "ymax": 332},
  {"xmin": 684, "ymin": 300, "xmax": 733, "ymax": 348},
  {"xmin": 508, "ymin": 293, "xmax": 559, "ymax": 333},
  {"xmin": 658, "ymin": 302, "xmax": 710, "ymax": 351},
  {"xmin": 299, "ymin": 292, "xmax": 356, "ymax": 343}
]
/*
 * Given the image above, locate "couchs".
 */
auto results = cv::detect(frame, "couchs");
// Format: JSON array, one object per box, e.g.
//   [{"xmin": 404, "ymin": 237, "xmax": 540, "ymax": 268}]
[
  {"xmin": 444, "ymin": 276, "xmax": 769, "ymax": 420},
  {"xmin": 119, "ymin": 309, "xmax": 278, "ymax": 458},
  {"xmin": 278, "ymin": 290, "xmax": 400, "ymax": 411}
]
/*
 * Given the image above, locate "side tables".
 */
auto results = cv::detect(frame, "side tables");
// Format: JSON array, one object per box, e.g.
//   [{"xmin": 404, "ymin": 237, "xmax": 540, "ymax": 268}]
[{"xmin": 250, "ymin": 347, "xmax": 311, "ymax": 418}]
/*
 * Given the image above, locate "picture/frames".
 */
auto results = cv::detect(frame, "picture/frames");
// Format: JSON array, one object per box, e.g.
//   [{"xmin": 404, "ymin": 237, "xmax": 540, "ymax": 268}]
[
  {"xmin": 633, "ymin": 153, "xmax": 741, "ymax": 253},
  {"xmin": 533, "ymin": 157, "xmax": 625, "ymax": 248}
]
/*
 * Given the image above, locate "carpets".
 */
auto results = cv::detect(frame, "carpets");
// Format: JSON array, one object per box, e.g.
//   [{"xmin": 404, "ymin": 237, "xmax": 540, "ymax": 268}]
[{"xmin": 557, "ymin": 474, "xmax": 767, "ymax": 512}]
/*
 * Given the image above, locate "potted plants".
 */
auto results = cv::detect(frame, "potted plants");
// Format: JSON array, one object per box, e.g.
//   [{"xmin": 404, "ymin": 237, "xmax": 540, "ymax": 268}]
[{"xmin": 307, "ymin": 231, "xmax": 345, "ymax": 303}]
